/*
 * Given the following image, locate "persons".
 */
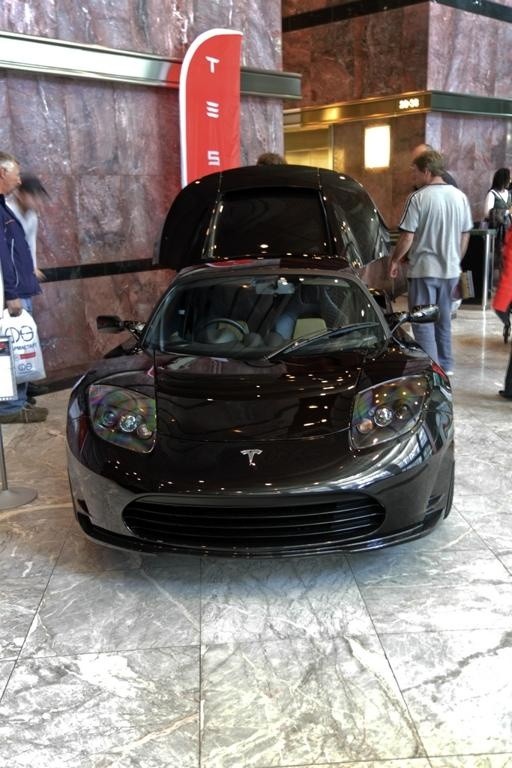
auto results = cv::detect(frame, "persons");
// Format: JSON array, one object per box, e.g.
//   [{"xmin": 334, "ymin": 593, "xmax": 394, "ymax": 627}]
[
  {"xmin": 257, "ymin": 153, "xmax": 285, "ymax": 165},
  {"xmin": 499, "ymin": 347, "xmax": 512, "ymax": 399},
  {"xmin": 0, "ymin": 151, "xmax": 49, "ymax": 424},
  {"xmin": 387, "ymin": 144, "xmax": 474, "ymax": 376},
  {"xmin": 484, "ymin": 168, "xmax": 512, "ymax": 343}
]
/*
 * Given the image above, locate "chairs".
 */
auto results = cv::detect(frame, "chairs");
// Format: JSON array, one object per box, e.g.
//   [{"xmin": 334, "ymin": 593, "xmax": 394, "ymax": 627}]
[
  {"xmin": 194, "ymin": 281, "xmax": 257, "ymax": 351},
  {"xmin": 267, "ymin": 284, "xmax": 350, "ymax": 349}
]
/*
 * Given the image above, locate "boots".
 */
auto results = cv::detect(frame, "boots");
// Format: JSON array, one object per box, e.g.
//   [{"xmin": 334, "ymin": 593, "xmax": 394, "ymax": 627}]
[
  {"xmin": 1, "ymin": 406, "xmax": 48, "ymax": 422},
  {"xmin": 499, "ymin": 391, "xmax": 512, "ymax": 398}
]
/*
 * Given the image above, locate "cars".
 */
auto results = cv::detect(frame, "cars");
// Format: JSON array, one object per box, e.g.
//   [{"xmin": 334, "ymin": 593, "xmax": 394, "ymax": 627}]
[{"xmin": 65, "ymin": 164, "xmax": 455, "ymax": 561}]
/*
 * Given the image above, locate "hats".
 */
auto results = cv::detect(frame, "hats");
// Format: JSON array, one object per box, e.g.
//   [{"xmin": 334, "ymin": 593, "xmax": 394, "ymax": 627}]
[{"xmin": 18, "ymin": 176, "xmax": 48, "ymax": 197}]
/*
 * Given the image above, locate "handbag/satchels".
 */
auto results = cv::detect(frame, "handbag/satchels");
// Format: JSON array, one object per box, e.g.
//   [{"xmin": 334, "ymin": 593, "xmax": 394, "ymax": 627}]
[{"xmin": 2, "ymin": 307, "xmax": 46, "ymax": 382}]
